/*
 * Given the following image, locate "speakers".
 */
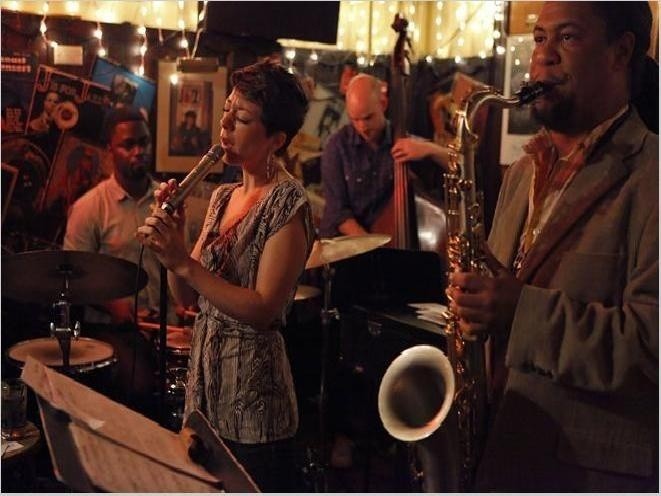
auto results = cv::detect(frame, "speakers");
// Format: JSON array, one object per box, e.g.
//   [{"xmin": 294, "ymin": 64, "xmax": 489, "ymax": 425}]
[{"xmin": 204, "ymin": 0, "xmax": 339, "ymax": 44}]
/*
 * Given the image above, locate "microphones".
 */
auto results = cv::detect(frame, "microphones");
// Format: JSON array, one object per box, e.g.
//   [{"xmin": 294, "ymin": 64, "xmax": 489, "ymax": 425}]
[{"xmin": 160, "ymin": 144, "xmax": 225, "ymax": 216}]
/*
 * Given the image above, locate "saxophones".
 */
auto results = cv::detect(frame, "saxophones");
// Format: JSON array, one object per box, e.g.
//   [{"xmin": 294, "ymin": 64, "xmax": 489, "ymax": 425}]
[{"xmin": 378, "ymin": 81, "xmax": 543, "ymax": 493}]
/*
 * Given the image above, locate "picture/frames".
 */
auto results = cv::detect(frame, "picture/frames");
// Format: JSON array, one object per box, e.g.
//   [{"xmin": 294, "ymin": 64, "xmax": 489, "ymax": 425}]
[
  {"xmin": 155, "ymin": 58, "xmax": 229, "ymax": 178},
  {"xmin": 499, "ymin": 30, "xmax": 542, "ymax": 166}
]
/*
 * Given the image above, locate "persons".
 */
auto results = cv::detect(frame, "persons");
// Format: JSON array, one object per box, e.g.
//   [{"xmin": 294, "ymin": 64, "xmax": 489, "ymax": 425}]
[
  {"xmin": 29, "ymin": 92, "xmax": 61, "ymax": 133},
  {"xmin": 170, "ymin": 111, "xmax": 205, "ymax": 156},
  {"xmin": 64, "ymin": 106, "xmax": 190, "ymax": 412},
  {"xmin": 317, "ymin": 73, "xmax": 463, "ymax": 240},
  {"xmin": 42, "ymin": 146, "xmax": 102, "ymax": 220},
  {"xmin": 445, "ymin": 1, "xmax": 659, "ymax": 493},
  {"xmin": 134, "ymin": 58, "xmax": 317, "ymax": 492}
]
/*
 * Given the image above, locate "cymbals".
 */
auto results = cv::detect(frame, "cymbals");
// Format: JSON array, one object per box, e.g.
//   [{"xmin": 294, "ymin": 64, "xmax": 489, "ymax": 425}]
[
  {"xmin": 293, "ymin": 284, "xmax": 319, "ymax": 300},
  {"xmin": 302, "ymin": 234, "xmax": 393, "ymax": 271},
  {"xmin": 1, "ymin": 248, "xmax": 148, "ymax": 304}
]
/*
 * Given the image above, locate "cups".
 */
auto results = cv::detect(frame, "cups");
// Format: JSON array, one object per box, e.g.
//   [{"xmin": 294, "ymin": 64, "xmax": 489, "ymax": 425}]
[{"xmin": 2, "ymin": 376, "xmax": 28, "ymax": 441}]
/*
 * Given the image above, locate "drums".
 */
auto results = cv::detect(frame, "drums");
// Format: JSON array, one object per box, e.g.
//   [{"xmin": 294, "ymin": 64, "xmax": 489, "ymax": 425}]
[
  {"xmin": 150, "ymin": 328, "xmax": 193, "ymax": 394},
  {"xmin": 9, "ymin": 335, "xmax": 119, "ymax": 400},
  {"xmin": 154, "ymin": 325, "xmax": 195, "ymax": 355}
]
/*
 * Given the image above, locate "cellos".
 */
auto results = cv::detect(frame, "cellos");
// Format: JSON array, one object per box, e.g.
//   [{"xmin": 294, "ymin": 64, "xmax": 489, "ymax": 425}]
[{"xmin": 370, "ymin": 14, "xmax": 447, "ymax": 254}]
